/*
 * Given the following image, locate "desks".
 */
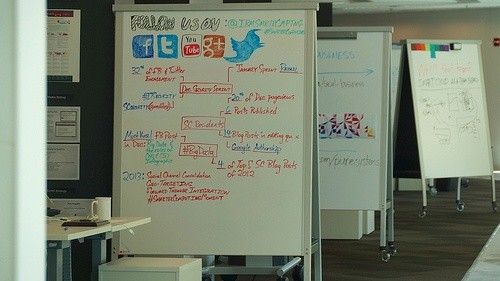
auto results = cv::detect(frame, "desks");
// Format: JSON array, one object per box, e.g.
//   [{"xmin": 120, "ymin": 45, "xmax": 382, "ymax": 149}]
[{"xmin": 45, "ymin": 215, "xmax": 151, "ymax": 249}]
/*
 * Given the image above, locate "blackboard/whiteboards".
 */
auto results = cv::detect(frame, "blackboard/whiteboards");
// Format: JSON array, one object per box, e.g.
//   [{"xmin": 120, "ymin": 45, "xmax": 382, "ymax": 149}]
[
  {"xmin": 405, "ymin": 37, "xmax": 495, "ymax": 179},
  {"xmin": 111, "ymin": 2, "xmax": 321, "ymax": 258},
  {"xmin": 317, "ymin": 27, "xmax": 393, "ymax": 212}
]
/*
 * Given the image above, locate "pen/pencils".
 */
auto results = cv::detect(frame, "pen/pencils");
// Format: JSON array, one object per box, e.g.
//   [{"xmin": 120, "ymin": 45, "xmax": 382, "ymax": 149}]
[{"xmin": 60, "ymin": 218, "xmax": 92, "ymax": 222}]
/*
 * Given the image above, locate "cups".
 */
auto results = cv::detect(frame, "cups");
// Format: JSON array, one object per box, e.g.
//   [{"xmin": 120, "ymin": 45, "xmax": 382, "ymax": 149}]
[{"xmin": 91, "ymin": 196, "xmax": 112, "ymax": 222}]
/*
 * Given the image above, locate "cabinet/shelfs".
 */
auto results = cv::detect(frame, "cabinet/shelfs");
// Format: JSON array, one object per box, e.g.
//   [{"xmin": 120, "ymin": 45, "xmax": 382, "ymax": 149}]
[{"xmin": 98, "ymin": 256, "xmax": 202, "ymax": 281}]
